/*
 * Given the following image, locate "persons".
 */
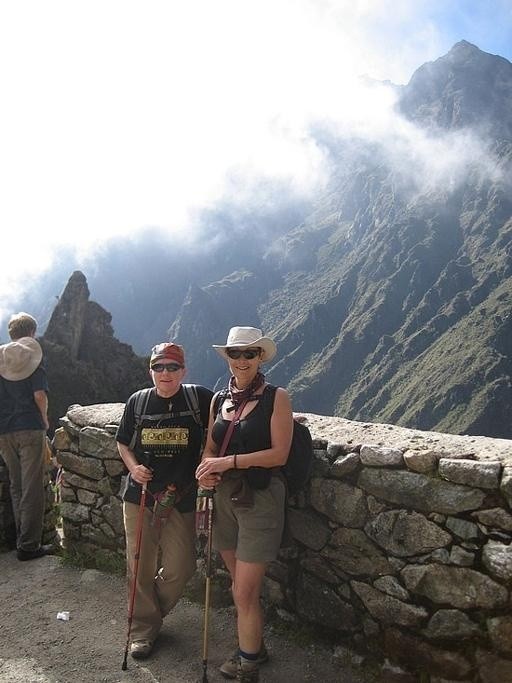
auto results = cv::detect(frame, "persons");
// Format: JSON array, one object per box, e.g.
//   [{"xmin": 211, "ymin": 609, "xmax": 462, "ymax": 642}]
[
  {"xmin": 115, "ymin": 343, "xmax": 215, "ymax": 659},
  {"xmin": 195, "ymin": 327, "xmax": 293, "ymax": 683},
  {"xmin": 0, "ymin": 312, "xmax": 55, "ymax": 561}
]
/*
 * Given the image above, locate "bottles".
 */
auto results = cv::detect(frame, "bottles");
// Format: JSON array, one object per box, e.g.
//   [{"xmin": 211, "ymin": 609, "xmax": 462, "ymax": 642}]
[
  {"xmin": 150, "ymin": 485, "xmax": 176, "ymax": 530},
  {"xmin": 195, "ymin": 484, "xmax": 213, "ymax": 538}
]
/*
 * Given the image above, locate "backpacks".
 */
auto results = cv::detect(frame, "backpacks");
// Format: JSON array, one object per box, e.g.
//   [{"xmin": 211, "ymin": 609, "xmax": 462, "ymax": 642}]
[{"xmin": 282, "ymin": 420, "xmax": 314, "ymax": 489}]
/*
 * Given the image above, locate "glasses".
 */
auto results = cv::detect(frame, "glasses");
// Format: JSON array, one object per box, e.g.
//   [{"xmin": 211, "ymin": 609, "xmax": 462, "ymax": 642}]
[
  {"xmin": 227, "ymin": 350, "xmax": 260, "ymax": 360},
  {"xmin": 151, "ymin": 363, "xmax": 184, "ymax": 372}
]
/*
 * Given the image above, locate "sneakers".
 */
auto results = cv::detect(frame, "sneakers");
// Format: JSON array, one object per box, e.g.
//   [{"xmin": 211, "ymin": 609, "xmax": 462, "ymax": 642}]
[
  {"xmin": 16, "ymin": 544, "xmax": 53, "ymax": 561},
  {"xmin": 219, "ymin": 636, "xmax": 269, "ymax": 683},
  {"xmin": 131, "ymin": 640, "xmax": 154, "ymax": 658}
]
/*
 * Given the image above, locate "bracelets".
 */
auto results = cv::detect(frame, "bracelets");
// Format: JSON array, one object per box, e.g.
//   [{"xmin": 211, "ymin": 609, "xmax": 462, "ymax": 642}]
[{"xmin": 234, "ymin": 453, "xmax": 237, "ymax": 472}]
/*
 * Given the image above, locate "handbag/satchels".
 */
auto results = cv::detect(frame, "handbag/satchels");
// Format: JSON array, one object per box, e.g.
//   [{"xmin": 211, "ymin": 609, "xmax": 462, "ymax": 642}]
[{"xmin": 43, "ymin": 435, "xmax": 51, "ymax": 465}]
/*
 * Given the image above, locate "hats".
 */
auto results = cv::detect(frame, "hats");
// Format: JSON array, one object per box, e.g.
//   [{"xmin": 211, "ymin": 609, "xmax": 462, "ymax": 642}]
[
  {"xmin": 0, "ymin": 337, "xmax": 43, "ymax": 382},
  {"xmin": 211, "ymin": 326, "xmax": 277, "ymax": 363},
  {"xmin": 150, "ymin": 343, "xmax": 184, "ymax": 364}
]
